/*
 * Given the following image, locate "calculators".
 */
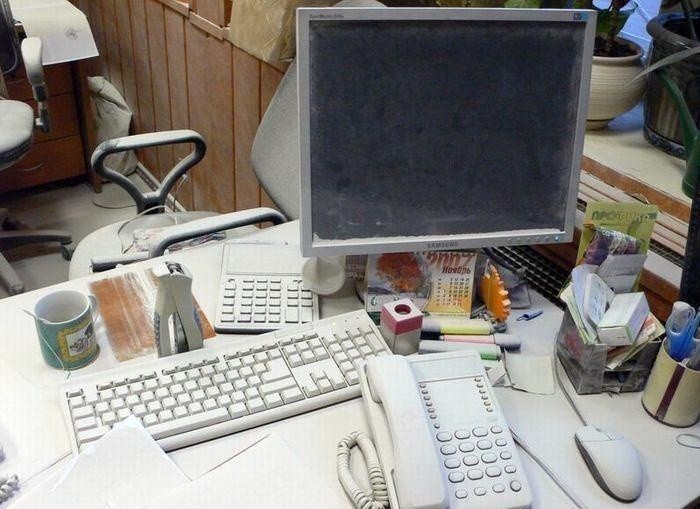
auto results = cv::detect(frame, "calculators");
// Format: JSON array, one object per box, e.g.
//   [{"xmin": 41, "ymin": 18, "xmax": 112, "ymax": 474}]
[{"xmin": 214, "ymin": 238, "xmax": 321, "ymax": 333}]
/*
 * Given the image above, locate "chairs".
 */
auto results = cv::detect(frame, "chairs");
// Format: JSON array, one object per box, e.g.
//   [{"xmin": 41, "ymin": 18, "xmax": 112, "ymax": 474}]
[
  {"xmin": 0, "ymin": 0, "xmax": 76, "ymax": 299},
  {"xmin": 68, "ymin": 0, "xmax": 390, "ymax": 283}
]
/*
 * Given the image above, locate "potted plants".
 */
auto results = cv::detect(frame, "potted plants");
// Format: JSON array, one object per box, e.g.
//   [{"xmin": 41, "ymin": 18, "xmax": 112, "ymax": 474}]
[{"xmin": 504, "ymin": 0, "xmax": 699, "ymax": 160}]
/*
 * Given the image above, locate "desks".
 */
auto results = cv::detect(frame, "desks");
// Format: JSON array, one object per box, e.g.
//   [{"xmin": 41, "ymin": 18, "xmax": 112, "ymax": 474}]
[{"xmin": 0, "ymin": 218, "xmax": 700, "ymax": 509}]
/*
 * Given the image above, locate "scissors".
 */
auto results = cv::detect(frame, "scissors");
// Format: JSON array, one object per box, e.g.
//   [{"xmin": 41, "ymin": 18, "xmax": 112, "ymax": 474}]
[{"xmin": 665, "ymin": 306, "xmax": 700, "ymax": 362}]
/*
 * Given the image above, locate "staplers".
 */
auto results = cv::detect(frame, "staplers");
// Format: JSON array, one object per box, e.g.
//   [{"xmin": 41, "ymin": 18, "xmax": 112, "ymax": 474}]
[{"xmin": 151, "ymin": 261, "xmax": 216, "ymax": 358}]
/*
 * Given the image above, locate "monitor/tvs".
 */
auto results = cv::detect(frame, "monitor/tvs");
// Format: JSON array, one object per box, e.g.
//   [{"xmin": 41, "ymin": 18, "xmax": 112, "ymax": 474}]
[{"xmin": 296, "ymin": 8, "xmax": 598, "ymax": 303}]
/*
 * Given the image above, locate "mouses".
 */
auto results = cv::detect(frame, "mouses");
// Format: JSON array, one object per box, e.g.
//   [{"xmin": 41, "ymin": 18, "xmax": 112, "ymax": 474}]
[{"xmin": 574, "ymin": 422, "xmax": 644, "ymax": 504}]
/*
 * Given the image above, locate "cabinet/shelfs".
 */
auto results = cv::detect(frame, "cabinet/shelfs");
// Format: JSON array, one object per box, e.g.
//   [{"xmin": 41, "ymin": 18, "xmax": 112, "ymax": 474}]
[{"xmin": 0, "ymin": 33, "xmax": 101, "ymax": 197}]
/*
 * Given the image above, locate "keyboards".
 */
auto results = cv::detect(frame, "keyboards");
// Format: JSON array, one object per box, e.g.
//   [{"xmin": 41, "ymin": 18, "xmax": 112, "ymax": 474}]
[{"xmin": 57, "ymin": 308, "xmax": 395, "ymax": 465}]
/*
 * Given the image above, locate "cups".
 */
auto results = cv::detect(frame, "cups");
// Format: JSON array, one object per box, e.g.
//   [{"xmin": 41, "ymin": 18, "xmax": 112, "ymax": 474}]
[
  {"xmin": 302, "ymin": 254, "xmax": 345, "ymax": 296},
  {"xmin": 34, "ymin": 291, "xmax": 100, "ymax": 369},
  {"xmin": 641, "ymin": 336, "xmax": 700, "ymax": 426}
]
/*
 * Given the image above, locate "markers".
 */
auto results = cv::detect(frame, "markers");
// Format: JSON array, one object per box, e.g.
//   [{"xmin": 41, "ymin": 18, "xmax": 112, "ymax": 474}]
[
  {"xmin": 418, "ymin": 319, "xmax": 521, "ymax": 360},
  {"xmin": 671, "ymin": 301, "xmax": 690, "ymax": 333}
]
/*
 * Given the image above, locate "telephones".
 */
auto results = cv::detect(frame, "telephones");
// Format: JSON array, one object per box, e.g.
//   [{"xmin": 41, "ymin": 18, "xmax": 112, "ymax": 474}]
[{"xmin": 357, "ymin": 350, "xmax": 532, "ymax": 509}]
